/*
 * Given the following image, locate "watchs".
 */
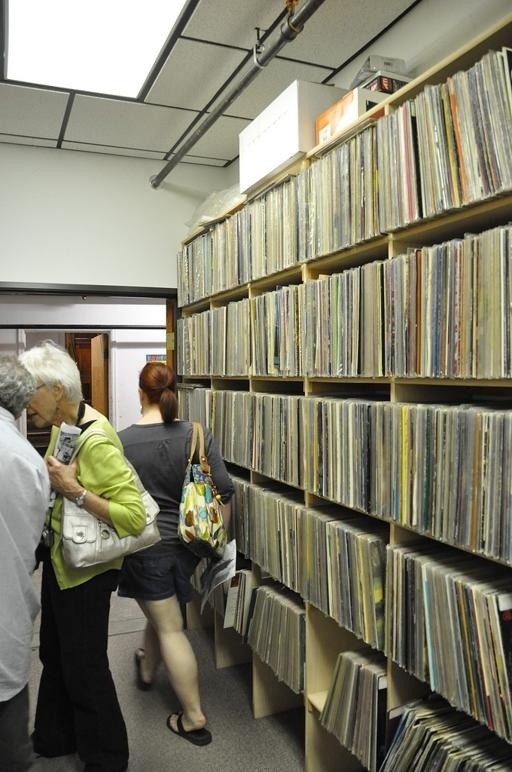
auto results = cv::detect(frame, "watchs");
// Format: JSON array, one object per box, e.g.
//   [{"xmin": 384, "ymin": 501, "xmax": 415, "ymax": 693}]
[{"xmin": 77, "ymin": 488, "xmax": 87, "ymax": 507}]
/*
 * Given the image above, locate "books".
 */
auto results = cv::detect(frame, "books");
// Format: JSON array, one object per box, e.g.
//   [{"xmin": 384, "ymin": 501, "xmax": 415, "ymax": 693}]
[
  {"xmin": 180, "ymin": 46, "xmax": 512, "ymax": 306},
  {"xmin": 236, "ymin": 571, "xmax": 512, "ymax": 772},
  {"xmin": 178, "ymin": 384, "xmax": 512, "ymax": 566},
  {"xmin": 179, "ymin": 225, "xmax": 511, "ymax": 380},
  {"xmin": 228, "ymin": 474, "xmax": 512, "ymax": 741}
]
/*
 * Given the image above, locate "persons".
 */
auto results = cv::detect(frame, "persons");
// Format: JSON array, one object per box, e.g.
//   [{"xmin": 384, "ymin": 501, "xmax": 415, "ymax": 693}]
[
  {"xmin": 1, "ymin": 356, "xmax": 52, "ymax": 772},
  {"xmin": 117, "ymin": 361, "xmax": 234, "ymax": 746},
  {"xmin": 21, "ymin": 341, "xmax": 148, "ymax": 770}
]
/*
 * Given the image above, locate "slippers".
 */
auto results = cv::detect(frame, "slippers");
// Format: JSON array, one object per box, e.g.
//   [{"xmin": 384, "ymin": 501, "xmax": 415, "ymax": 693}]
[
  {"xmin": 134, "ymin": 647, "xmax": 154, "ymax": 692},
  {"xmin": 167, "ymin": 713, "xmax": 213, "ymax": 747}
]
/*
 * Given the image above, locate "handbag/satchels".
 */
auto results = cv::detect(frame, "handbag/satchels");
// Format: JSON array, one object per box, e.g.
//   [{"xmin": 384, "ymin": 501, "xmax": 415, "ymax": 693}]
[
  {"xmin": 60, "ymin": 429, "xmax": 163, "ymax": 571},
  {"xmin": 177, "ymin": 421, "xmax": 228, "ymax": 561}
]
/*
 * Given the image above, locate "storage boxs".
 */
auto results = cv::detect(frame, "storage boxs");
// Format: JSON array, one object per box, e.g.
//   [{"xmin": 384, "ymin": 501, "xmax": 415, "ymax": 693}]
[{"xmin": 235, "ymin": 55, "xmax": 425, "ymax": 196}]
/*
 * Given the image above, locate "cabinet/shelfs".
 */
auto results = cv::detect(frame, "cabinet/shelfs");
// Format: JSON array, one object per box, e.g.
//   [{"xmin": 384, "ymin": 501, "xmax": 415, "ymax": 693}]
[{"xmin": 166, "ymin": 8, "xmax": 512, "ymax": 770}]
